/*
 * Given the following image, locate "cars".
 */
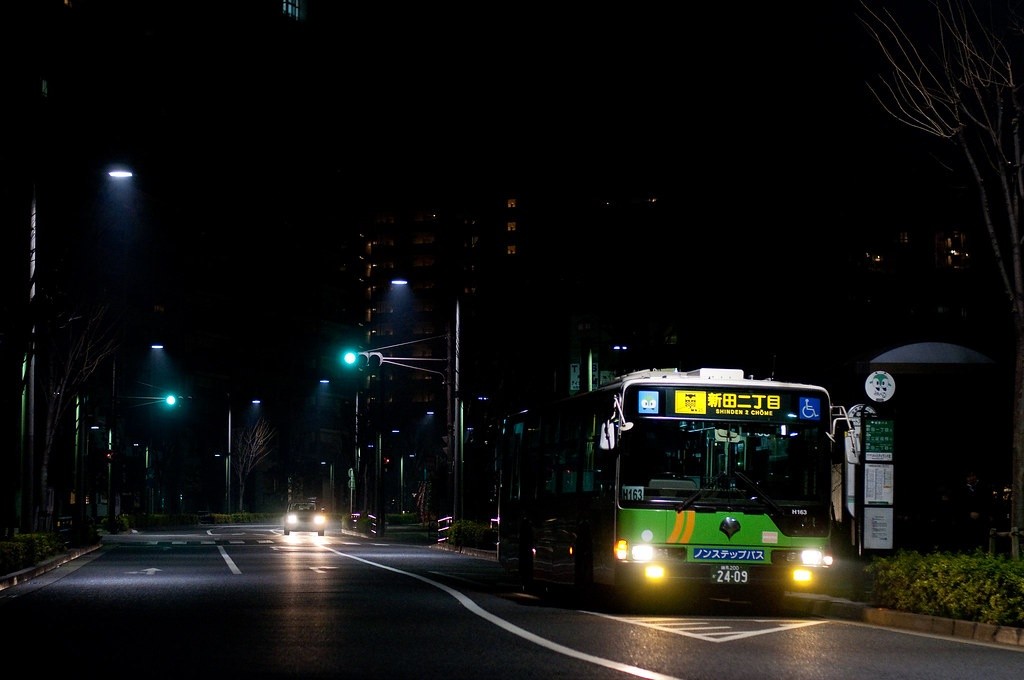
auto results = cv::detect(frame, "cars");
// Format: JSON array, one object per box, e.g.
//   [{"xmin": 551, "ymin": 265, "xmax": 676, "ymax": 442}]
[{"xmin": 283, "ymin": 500, "xmax": 326, "ymax": 535}]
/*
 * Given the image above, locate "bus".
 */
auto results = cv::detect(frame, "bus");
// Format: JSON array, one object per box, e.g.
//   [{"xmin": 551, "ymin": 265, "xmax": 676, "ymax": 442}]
[{"xmin": 495, "ymin": 366, "xmax": 859, "ymax": 618}]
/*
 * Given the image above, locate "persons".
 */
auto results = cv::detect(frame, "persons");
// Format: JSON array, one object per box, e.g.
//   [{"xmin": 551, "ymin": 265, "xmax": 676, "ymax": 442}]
[{"xmin": 962, "ymin": 471, "xmax": 990, "ymax": 555}]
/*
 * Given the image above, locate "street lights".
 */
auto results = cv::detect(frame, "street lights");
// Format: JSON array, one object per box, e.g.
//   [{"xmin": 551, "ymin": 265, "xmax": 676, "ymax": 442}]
[
  {"xmin": 133, "ymin": 443, "xmax": 149, "ymax": 522},
  {"xmin": 320, "ymin": 378, "xmax": 359, "ymax": 470},
  {"xmin": 390, "ymin": 275, "xmax": 462, "ymax": 527},
  {"xmin": 322, "ymin": 461, "xmax": 334, "ymax": 513},
  {"xmin": 90, "ymin": 426, "xmax": 113, "ymax": 523},
  {"xmin": 226, "ymin": 395, "xmax": 262, "ymax": 514}
]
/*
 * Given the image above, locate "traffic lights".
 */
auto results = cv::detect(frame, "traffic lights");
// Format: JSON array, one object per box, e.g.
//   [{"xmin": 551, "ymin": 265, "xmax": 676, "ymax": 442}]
[
  {"xmin": 344, "ymin": 352, "xmax": 385, "ymax": 369},
  {"xmin": 165, "ymin": 396, "xmax": 195, "ymax": 407}
]
